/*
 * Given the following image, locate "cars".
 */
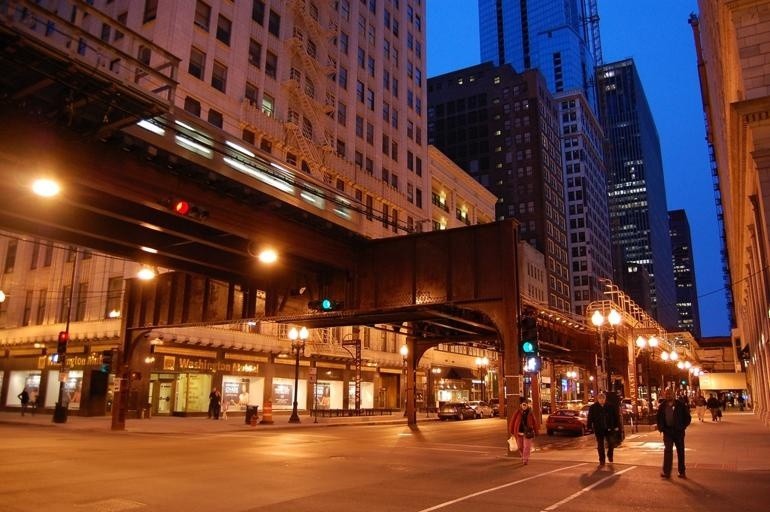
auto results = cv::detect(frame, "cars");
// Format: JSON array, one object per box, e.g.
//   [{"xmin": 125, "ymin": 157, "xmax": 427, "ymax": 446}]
[
  {"xmin": 546, "ymin": 409, "xmax": 589, "ymax": 436},
  {"xmin": 438, "ymin": 392, "xmax": 695, "ymax": 424}
]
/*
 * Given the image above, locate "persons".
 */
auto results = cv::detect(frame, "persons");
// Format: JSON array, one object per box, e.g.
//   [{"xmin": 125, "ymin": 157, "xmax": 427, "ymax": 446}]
[
  {"xmin": 16, "ymin": 388, "xmax": 29, "ymax": 418},
  {"xmin": 316, "ymin": 386, "xmax": 330, "ymax": 409},
  {"xmin": 238, "ymin": 385, "xmax": 248, "ymax": 408},
  {"xmin": 508, "ymin": 396, "xmax": 539, "ymax": 467},
  {"xmin": 665, "ymin": 390, "xmax": 746, "ymax": 426},
  {"xmin": 62, "ymin": 386, "xmax": 71, "ymax": 421},
  {"xmin": 588, "ymin": 391, "xmax": 620, "ymax": 469},
  {"xmin": 655, "ymin": 389, "xmax": 692, "ymax": 478},
  {"xmin": 206, "ymin": 385, "xmax": 229, "ymax": 420}
]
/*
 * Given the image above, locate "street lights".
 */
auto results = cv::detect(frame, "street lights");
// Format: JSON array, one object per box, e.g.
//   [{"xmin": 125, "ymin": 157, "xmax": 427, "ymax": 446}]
[
  {"xmin": 475, "ymin": 356, "xmax": 489, "ymax": 401},
  {"xmin": 288, "ymin": 326, "xmax": 309, "ymax": 424},
  {"xmin": 591, "ymin": 308, "xmax": 620, "ymax": 393},
  {"xmin": 677, "ymin": 357, "xmax": 691, "ymax": 396},
  {"xmin": 400, "ymin": 345, "xmax": 409, "ymax": 417},
  {"xmin": 661, "ymin": 350, "xmax": 678, "ymax": 390},
  {"xmin": 636, "ymin": 335, "xmax": 658, "ymax": 425},
  {"xmin": 566, "ymin": 371, "xmax": 576, "ymax": 400},
  {"xmin": 690, "ymin": 368, "xmax": 704, "ymax": 398}
]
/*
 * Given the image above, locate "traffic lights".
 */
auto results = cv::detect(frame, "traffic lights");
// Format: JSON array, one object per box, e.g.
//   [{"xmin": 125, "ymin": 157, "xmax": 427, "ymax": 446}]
[
  {"xmin": 161, "ymin": 194, "xmax": 209, "ymax": 222},
  {"xmin": 102, "ymin": 349, "xmax": 118, "ymax": 374},
  {"xmin": 681, "ymin": 377, "xmax": 687, "ymax": 386},
  {"xmin": 130, "ymin": 372, "xmax": 141, "ymax": 382},
  {"xmin": 307, "ymin": 297, "xmax": 344, "ymax": 312},
  {"xmin": 520, "ymin": 310, "xmax": 539, "ymax": 358},
  {"xmin": 58, "ymin": 331, "xmax": 68, "ymax": 355},
  {"xmin": 526, "ymin": 357, "xmax": 542, "ymax": 371}
]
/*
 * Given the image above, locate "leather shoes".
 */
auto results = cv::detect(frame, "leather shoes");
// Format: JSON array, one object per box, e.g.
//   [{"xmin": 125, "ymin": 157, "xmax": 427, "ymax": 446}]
[
  {"xmin": 678, "ymin": 471, "xmax": 685, "ymax": 478},
  {"xmin": 606, "ymin": 454, "xmax": 614, "ymax": 462},
  {"xmin": 661, "ymin": 473, "xmax": 669, "ymax": 479}
]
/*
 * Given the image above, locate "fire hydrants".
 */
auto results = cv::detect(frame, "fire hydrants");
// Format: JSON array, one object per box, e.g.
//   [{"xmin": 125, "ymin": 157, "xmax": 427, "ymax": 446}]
[{"xmin": 249, "ymin": 414, "xmax": 260, "ymax": 426}]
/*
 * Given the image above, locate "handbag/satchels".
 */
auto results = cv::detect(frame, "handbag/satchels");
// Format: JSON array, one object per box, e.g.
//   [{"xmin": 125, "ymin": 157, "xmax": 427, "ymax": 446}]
[
  {"xmin": 507, "ymin": 436, "xmax": 518, "ymax": 452},
  {"xmin": 524, "ymin": 428, "xmax": 535, "ymax": 439}
]
